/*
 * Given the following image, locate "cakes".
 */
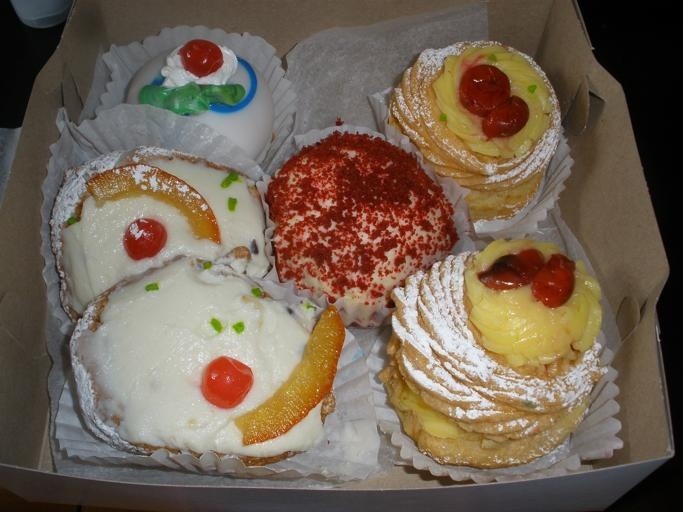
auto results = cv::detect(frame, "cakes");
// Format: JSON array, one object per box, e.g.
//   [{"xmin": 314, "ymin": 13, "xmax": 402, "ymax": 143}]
[
  {"xmin": 120, "ymin": 36, "xmax": 276, "ymax": 164},
  {"xmin": 386, "ymin": 38, "xmax": 562, "ymax": 227},
  {"xmin": 263, "ymin": 132, "xmax": 461, "ymax": 327},
  {"xmin": 374, "ymin": 237, "xmax": 608, "ymax": 471}
]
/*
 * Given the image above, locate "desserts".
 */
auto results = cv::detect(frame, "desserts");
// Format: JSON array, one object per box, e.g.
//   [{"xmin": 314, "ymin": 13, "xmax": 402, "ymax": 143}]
[{"xmin": 47, "ymin": 146, "xmax": 347, "ymax": 470}]
